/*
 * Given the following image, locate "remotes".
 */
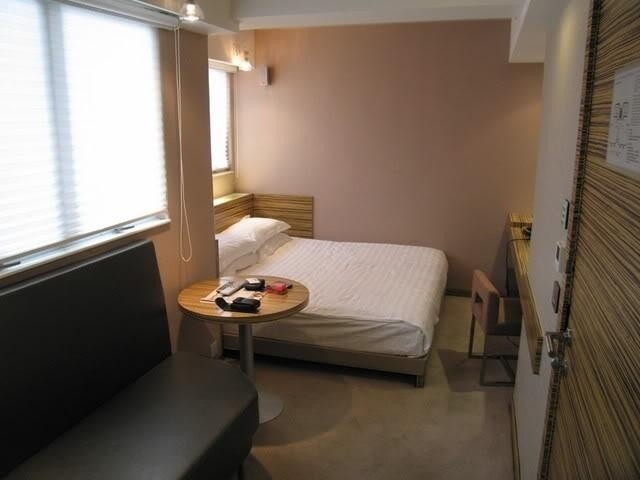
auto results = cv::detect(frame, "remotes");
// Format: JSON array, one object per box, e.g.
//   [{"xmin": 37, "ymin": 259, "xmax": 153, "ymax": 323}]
[
  {"xmin": 220, "ymin": 280, "xmax": 248, "ymax": 296},
  {"xmin": 216, "ymin": 281, "xmax": 233, "ymax": 293}
]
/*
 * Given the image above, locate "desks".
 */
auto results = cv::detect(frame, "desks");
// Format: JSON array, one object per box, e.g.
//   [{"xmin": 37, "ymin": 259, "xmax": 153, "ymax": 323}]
[
  {"xmin": 507, "ymin": 213, "xmax": 534, "ymax": 272},
  {"xmin": 178, "ymin": 276, "xmax": 310, "ymax": 424}
]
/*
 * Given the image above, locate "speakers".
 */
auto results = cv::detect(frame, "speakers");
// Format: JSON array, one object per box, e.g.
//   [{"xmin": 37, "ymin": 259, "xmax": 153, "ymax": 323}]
[{"xmin": 256, "ymin": 64, "xmax": 268, "ymax": 87}]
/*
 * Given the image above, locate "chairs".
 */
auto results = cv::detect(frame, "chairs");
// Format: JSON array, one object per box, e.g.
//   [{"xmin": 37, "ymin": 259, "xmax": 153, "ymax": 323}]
[{"xmin": 468, "ymin": 271, "xmax": 521, "ymax": 387}]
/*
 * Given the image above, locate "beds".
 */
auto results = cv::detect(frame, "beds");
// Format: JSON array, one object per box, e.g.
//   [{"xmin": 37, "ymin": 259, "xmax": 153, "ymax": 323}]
[{"xmin": 214, "ymin": 192, "xmax": 448, "ymax": 387}]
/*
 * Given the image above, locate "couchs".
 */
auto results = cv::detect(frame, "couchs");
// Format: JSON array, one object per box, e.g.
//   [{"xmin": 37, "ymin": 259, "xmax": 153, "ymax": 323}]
[{"xmin": 0, "ymin": 239, "xmax": 259, "ymax": 480}]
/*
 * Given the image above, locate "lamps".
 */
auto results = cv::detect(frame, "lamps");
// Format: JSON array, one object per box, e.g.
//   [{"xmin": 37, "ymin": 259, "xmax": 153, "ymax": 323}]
[
  {"xmin": 239, "ymin": 57, "xmax": 251, "ymax": 71},
  {"xmin": 178, "ymin": 0, "xmax": 204, "ymax": 21}
]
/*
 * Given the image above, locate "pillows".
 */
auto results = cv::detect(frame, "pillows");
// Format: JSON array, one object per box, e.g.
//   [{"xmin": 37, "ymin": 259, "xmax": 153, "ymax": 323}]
[{"xmin": 214, "ymin": 215, "xmax": 293, "ymax": 276}]
